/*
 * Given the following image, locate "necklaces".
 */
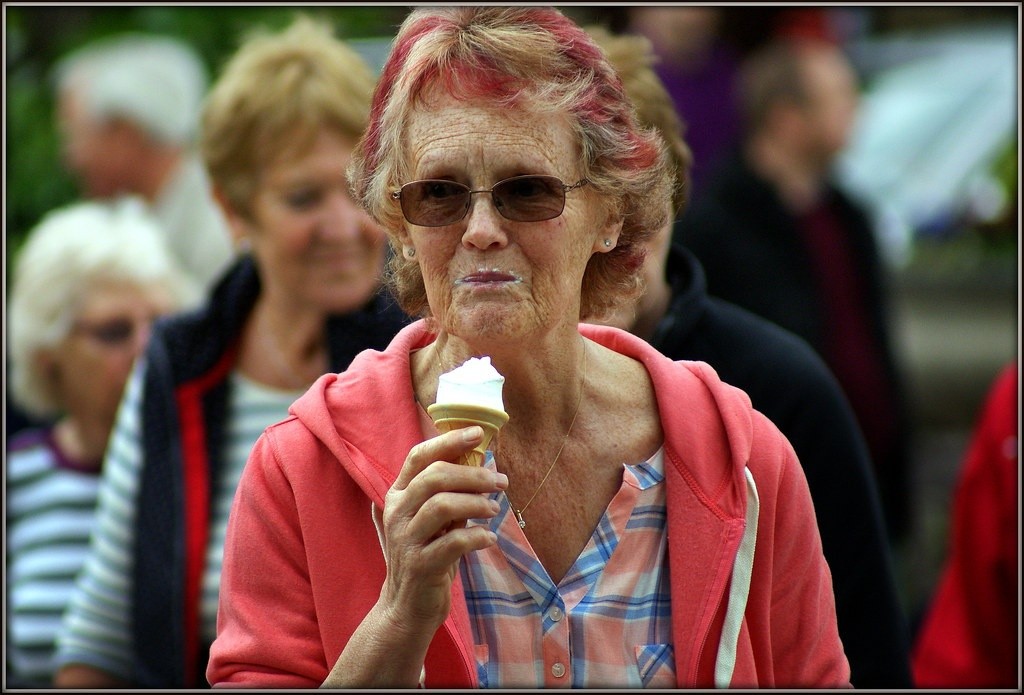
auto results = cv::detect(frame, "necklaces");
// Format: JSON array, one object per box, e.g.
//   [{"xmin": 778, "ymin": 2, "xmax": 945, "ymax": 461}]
[{"xmin": 431, "ymin": 333, "xmax": 587, "ymax": 529}]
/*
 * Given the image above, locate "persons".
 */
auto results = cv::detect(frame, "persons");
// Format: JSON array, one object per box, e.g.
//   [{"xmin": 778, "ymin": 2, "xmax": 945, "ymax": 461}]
[
  {"xmin": 8, "ymin": 191, "xmax": 204, "ymax": 688},
  {"xmin": 55, "ymin": 15, "xmax": 423, "ymax": 688},
  {"xmin": 205, "ymin": 5, "xmax": 853, "ymax": 690},
  {"xmin": 48, "ymin": 37, "xmax": 238, "ymax": 288},
  {"xmin": 681, "ymin": 38, "xmax": 919, "ymax": 554},
  {"xmin": 624, "ymin": 8, "xmax": 745, "ymax": 208},
  {"xmin": 566, "ymin": 27, "xmax": 911, "ymax": 690}
]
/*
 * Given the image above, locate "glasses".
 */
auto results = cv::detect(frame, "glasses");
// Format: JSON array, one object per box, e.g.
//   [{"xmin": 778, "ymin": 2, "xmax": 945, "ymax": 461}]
[
  {"xmin": 71, "ymin": 311, "xmax": 138, "ymax": 343},
  {"xmin": 392, "ymin": 174, "xmax": 589, "ymax": 228}
]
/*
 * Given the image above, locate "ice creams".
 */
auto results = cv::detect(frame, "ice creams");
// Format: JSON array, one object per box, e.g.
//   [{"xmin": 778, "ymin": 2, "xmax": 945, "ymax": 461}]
[{"xmin": 427, "ymin": 355, "xmax": 506, "ymax": 468}]
